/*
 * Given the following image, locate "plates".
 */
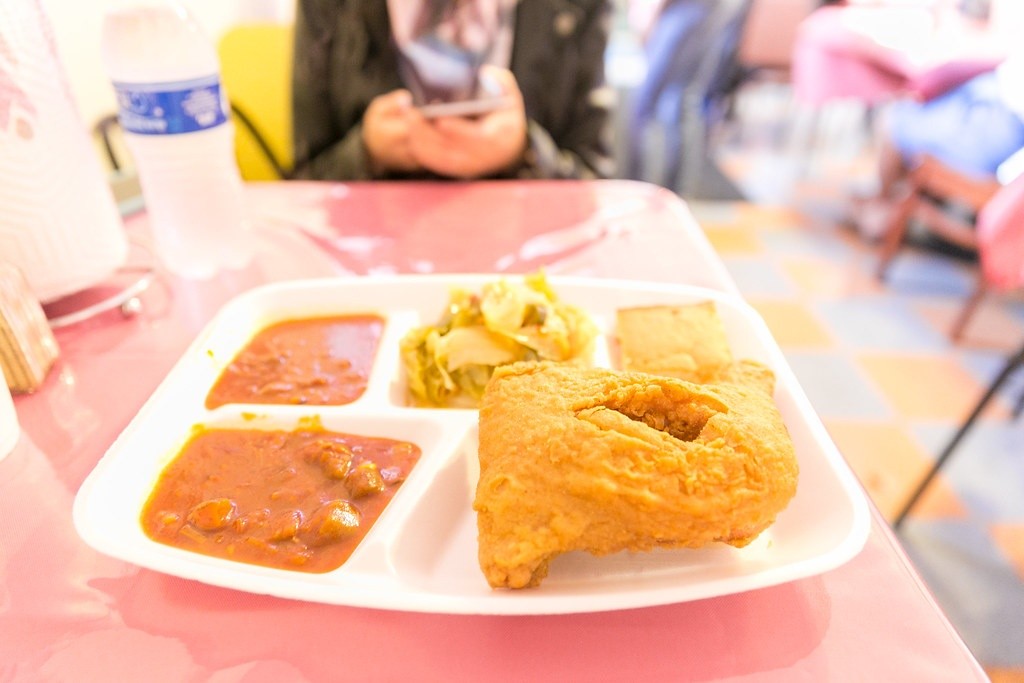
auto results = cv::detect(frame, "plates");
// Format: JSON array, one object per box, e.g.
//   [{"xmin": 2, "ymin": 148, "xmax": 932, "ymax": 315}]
[{"xmin": 71, "ymin": 274, "xmax": 872, "ymax": 616}]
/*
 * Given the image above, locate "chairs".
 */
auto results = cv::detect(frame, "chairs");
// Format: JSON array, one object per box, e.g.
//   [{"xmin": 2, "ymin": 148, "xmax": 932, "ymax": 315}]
[
  {"xmin": 219, "ymin": 23, "xmax": 297, "ymax": 181},
  {"xmin": 873, "ymin": 154, "xmax": 1023, "ymax": 339}
]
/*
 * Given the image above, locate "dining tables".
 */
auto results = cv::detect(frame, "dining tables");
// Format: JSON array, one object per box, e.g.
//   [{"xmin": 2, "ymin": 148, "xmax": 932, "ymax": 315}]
[{"xmin": 0, "ymin": 177, "xmax": 993, "ymax": 683}]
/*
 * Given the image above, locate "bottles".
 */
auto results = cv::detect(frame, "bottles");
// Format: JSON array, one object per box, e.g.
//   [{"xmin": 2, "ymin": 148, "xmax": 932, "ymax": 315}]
[{"xmin": 102, "ymin": 0, "xmax": 256, "ymax": 278}]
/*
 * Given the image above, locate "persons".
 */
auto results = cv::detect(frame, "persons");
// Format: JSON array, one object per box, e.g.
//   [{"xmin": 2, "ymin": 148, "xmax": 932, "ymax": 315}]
[
  {"xmin": 294, "ymin": 1, "xmax": 617, "ymax": 181},
  {"xmin": 627, "ymin": 0, "xmax": 1024, "ymax": 241}
]
